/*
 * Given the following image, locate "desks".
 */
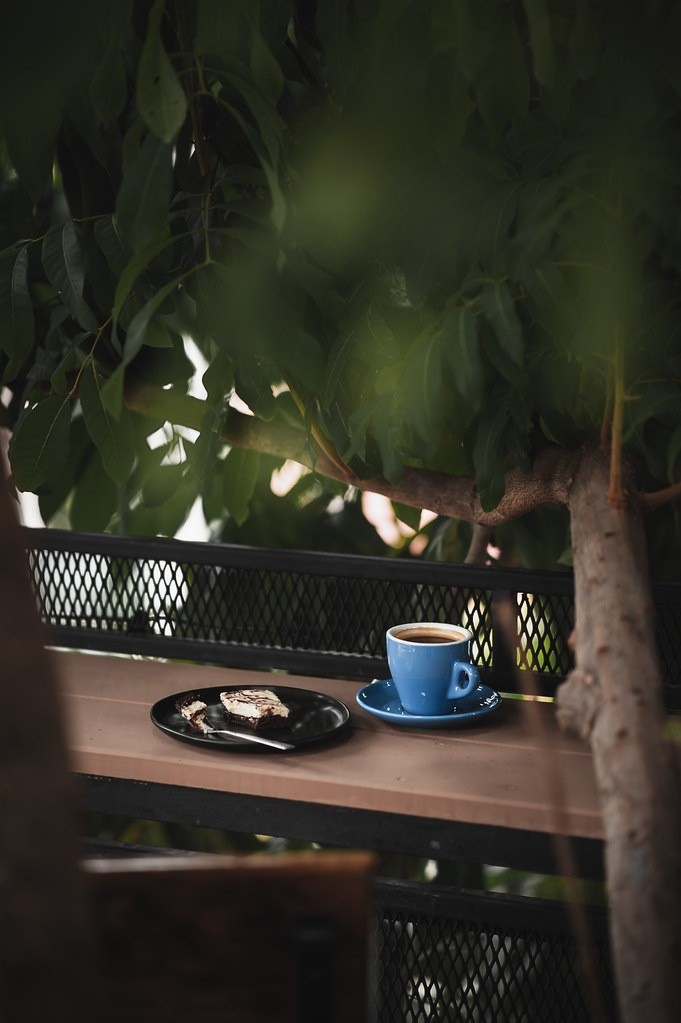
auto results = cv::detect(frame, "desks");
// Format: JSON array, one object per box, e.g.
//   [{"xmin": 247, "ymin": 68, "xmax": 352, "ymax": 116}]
[{"xmin": 46, "ymin": 648, "xmax": 607, "ymax": 840}]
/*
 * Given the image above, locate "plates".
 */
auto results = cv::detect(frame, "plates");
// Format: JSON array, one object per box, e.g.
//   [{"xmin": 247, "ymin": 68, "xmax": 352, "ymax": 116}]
[
  {"xmin": 149, "ymin": 685, "xmax": 349, "ymax": 755},
  {"xmin": 356, "ymin": 678, "xmax": 503, "ymax": 726}
]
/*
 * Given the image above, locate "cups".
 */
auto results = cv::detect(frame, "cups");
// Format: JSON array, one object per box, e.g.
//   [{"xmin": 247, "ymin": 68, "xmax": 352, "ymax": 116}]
[{"xmin": 386, "ymin": 623, "xmax": 474, "ymax": 716}]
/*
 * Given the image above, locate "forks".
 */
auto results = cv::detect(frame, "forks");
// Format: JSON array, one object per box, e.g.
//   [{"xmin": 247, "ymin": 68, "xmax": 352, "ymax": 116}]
[{"xmin": 176, "ymin": 699, "xmax": 296, "ymax": 750}]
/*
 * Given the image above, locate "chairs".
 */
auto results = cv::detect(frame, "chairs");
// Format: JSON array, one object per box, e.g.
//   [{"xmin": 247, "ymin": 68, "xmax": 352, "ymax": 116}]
[{"xmin": 0, "ymin": 430, "xmax": 371, "ymax": 1023}]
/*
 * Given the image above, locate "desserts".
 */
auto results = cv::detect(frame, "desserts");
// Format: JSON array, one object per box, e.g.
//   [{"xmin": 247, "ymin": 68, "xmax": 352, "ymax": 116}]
[
  {"xmin": 220, "ymin": 690, "xmax": 291, "ymax": 732},
  {"xmin": 174, "ymin": 692, "xmax": 214, "ymax": 733}
]
